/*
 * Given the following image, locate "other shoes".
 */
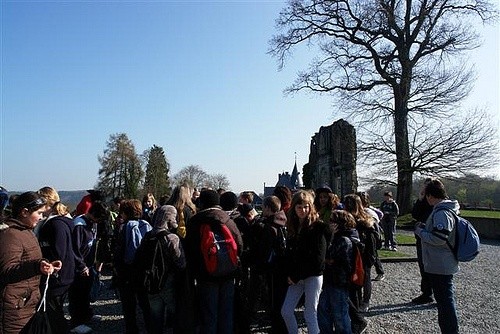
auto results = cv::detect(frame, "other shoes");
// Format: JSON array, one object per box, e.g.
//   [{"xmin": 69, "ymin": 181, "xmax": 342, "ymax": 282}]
[
  {"xmin": 359, "ymin": 302, "xmax": 369, "ymax": 312},
  {"xmin": 412, "ymin": 294, "xmax": 435, "ymax": 304},
  {"xmin": 90, "ymin": 314, "xmax": 102, "ymax": 321},
  {"xmin": 357, "ymin": 319, "xmax": 369, "ymax": 334},
  {"xmin": 384, "ymin": 246, "xmax": 398, "ymax": 251},
  {"xmin": 69, "ymin": 324, "xmax": 92, "ymax": 334},
  {"xmin": 375, "ymin": 274, "xmax": 386, "ymax": 281}
]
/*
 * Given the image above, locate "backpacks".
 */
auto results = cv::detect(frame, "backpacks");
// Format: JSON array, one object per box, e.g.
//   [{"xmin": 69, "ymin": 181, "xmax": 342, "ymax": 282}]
[
  {"xmin": 431, "ymin": 207, "xmax": 481, "ymax": 262},
  {"xmin": 270, "ymin": 223, "xmax": 293, "ymax": 266},
  {"xmin": 194, "ymin": 217, "xmax": 238, "ymax": 283},
  {"xmin": 121, "ymin": 219, "xmax": 153, "ymax": 264},
  {"xmin": 129, "ymin": 230, "xmax": 170, "ymax": 295}
]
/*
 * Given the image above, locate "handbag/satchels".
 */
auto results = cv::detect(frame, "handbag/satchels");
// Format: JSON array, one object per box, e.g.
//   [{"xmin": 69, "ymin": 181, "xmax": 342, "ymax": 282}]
[
  {"xmin": 342, "ymin": 236, "xmax": 364, "ymax": 288},
  {"xmin": 372, "ymin": 230, "xmax": 382, "ymax": 250}
]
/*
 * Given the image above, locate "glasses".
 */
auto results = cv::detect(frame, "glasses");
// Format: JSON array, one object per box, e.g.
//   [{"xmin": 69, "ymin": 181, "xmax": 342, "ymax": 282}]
[{"xmin": 27, "ymin": 196, "xmax": 47, "ymax": 207}]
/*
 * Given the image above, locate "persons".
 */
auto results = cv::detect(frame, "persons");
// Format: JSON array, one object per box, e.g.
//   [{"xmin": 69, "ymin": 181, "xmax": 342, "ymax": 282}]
[
  {"xmin": 403, "ymin": 179, "xmax": 460, "ymax": 334},
  {"xmin": 380, "ymin": 191, "xmax": 399, "ymax": 251},
  {"xmin": 140, "ymin": 205, "xmax": 181, "ymax": 334},
  {"xmin": 185, "ymin": 190, "xmax": 243, "ymax": 334},
  {"xmin": 412, "ymin": 178, "xmax": 437, "ymax": 303},
  {"xmin": 0, "ymin": 183, "xmax": 383, "ymax": 334},
  {"xmin": 281, "ymin": 190, "xmax": 332, "ymax": 334},
  {"xmin": 0, "ymin": 191, "xmax": 62, "ymax": 334},
  {"xmin": 317, "ymin": 209, "xmax": 361, "ymax": 334}
]
